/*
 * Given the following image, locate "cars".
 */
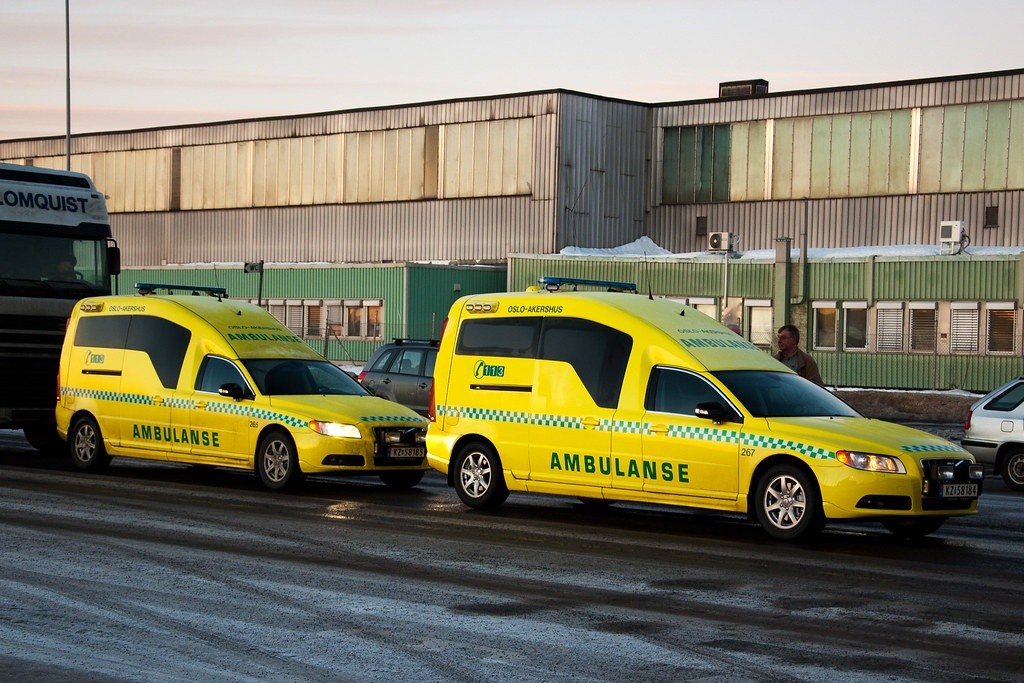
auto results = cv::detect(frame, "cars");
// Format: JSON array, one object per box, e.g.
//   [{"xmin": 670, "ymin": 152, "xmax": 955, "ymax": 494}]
[{"xmin": 957, "ymin": 373, "xmax": 1024, "ymax": 492}]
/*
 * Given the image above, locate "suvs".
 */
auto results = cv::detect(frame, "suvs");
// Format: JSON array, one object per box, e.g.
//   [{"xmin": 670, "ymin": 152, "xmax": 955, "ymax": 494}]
[{"xmin": 353, "ymin": 334, "xmax": 442, "ymax": 419}]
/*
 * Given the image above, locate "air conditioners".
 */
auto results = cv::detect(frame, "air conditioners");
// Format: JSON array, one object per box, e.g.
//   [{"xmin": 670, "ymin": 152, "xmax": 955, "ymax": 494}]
[
  {"xmin": 708, "ymin": 231, "xmax": 732, "ymax": 251},
  {"xmin": 939, "ymin": 220, "xmax": 966, "ymax": 243}
]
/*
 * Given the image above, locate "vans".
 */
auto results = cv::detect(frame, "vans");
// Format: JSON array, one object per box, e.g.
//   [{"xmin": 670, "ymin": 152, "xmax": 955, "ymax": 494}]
[
  {"xmin": 426, "ymin": 290, "xmax": 988, "ymax": 547},
  {"xmin": 56, "ymin": 281, "xmax": 436, "ymax": 499}
]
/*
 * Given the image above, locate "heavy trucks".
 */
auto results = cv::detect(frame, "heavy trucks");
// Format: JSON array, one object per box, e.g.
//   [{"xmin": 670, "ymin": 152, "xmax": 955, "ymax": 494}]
[{"xmin": 0, "ymin": 162, "xmax": 122, "ymax": 458}]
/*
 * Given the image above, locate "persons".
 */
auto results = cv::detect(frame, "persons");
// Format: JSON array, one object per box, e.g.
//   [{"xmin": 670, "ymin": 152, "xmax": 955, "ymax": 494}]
[
  {"xmin": 727, "ymin": 324, "xmax": 742, "ymax": 335},
  {"xmin": 770, "ymin": 325, "xmax": 826, "ymax": 388}
]
